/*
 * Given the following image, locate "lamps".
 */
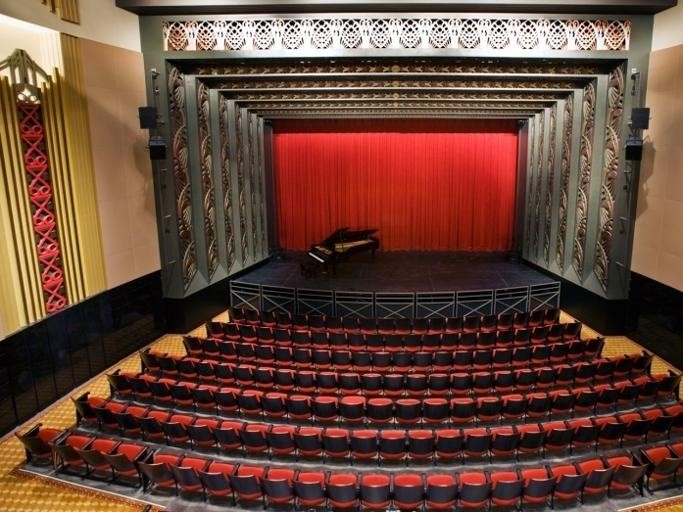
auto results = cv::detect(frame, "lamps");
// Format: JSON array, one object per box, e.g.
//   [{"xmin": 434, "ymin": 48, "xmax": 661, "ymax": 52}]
[
  {"xmin": 625, "ymin": 107, "xmax": 650, "ymax": 161},
  {"xmin": 138, "ymin": 106, "xmax": 168, "ymax": 160}
]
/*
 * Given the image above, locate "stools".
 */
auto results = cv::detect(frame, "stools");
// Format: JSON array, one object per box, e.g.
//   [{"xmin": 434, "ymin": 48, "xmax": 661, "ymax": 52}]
[{"xmin": 299, "ymin": 262, "xmax": 318, "ymax": 280}]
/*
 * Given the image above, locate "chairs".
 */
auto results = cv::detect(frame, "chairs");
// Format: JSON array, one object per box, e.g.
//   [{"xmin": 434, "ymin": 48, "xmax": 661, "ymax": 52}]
[{"xmin": 12, "ymin": 306, "xmax": 683, "ymax": 512}]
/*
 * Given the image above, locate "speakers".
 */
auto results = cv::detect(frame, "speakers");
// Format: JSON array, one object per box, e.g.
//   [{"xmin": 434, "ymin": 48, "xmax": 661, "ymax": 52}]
[
  {"xmin": 626, "ymin": 140, "xmax": 643, "ymax": 161},
  {"xmin": 631, "ymin": 107, "xmax": 650, "ymax": 129},
  {"xmin": 148, "ymin": 143, "xmax": 168, "ymax": 162},
  {"xmin": 138, "ymin": 105, "xmax": 156, "ymax": 130}
]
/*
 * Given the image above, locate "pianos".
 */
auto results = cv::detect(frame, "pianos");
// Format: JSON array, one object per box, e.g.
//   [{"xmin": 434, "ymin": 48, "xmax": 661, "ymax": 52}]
[{"xmin": 303, "ymin": 228, "xmax": 379, "ymax": 277}]
